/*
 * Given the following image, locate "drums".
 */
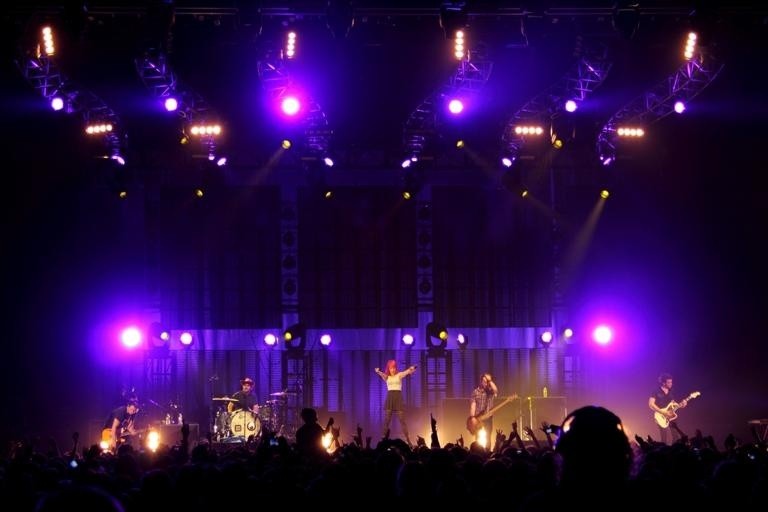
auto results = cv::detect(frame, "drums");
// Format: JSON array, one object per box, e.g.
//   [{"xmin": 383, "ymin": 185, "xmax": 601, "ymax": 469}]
[{"xmin": 215, "ymin": 404, "xmax": 282, "ymax": 439}]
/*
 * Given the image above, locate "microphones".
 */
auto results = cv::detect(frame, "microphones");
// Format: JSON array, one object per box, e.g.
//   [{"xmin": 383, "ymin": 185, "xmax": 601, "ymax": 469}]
[{"xmin": 414, "ymin": 364, "xmax": 418, "ymax": 369}]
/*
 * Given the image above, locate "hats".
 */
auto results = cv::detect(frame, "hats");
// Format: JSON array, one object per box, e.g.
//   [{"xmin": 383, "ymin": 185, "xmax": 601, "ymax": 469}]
[{"xmin": 240, "ymin": 378, "xmax": 255, "ymax": 387}]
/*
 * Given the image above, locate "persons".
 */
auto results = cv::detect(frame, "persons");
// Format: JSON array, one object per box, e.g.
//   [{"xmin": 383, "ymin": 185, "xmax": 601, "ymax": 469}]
[
  {"xmin": 648, "ymin": 374, "xmax": 687, "ymax": 441},
  {"xmin": 99, "ymin": 402, "xmax": 142, "ymax": 448},
  {"xmin": 0, "ymin": 408, "xmax": 768, "ymax": 512},
  {"xmin": 469, "ymin": 372, "xmax": 499, "ymax": 449},
  {"xmin": 228, "ymin": 377, "xmax": 260, "ymax": 414},
  {"xmin": 373, "ymin": 359, "xmax": 416, "ymax": 446}
]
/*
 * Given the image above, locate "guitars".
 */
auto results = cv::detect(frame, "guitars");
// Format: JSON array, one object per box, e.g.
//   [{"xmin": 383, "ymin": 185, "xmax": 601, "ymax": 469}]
[
  {"xmin": 654, "ymin": 391, "xmax": 701, "ymax": 429},
  {"xmin": 102, "ymin": 424, "xmax": 154, "ymax": 445},
  {"xmin": 467, "ymin": 394, "xmax": 518, "ymax": 436}
]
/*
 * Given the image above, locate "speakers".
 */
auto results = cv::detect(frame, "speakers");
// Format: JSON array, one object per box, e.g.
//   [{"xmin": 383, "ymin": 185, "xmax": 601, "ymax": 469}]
[{"xmin": 523, "ymin": 396, "xmax": 565, "ymax": 441}]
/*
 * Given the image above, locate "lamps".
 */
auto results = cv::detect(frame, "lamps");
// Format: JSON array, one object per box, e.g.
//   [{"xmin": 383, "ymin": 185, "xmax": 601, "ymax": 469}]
[
  {"xmin": 424, "ymin": 320, "xmax": 449, "ymax": 354},
  {"xmin": 283, "ymin": 323, "xmax": 308, "ymax": 354}
]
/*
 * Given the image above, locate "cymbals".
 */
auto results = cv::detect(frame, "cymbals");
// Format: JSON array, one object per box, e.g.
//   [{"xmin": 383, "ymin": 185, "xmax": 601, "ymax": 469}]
[
  {"xmin": 270, "ymin": 392, "xmax": 294, "ymax": 396},
  {"xmin": 213, "ymin": 397, "xmax": 239, "ymax": 401},
  {"xmin": 266, "ymin": 400, "xmax": 285, "ymax": 404}
]
